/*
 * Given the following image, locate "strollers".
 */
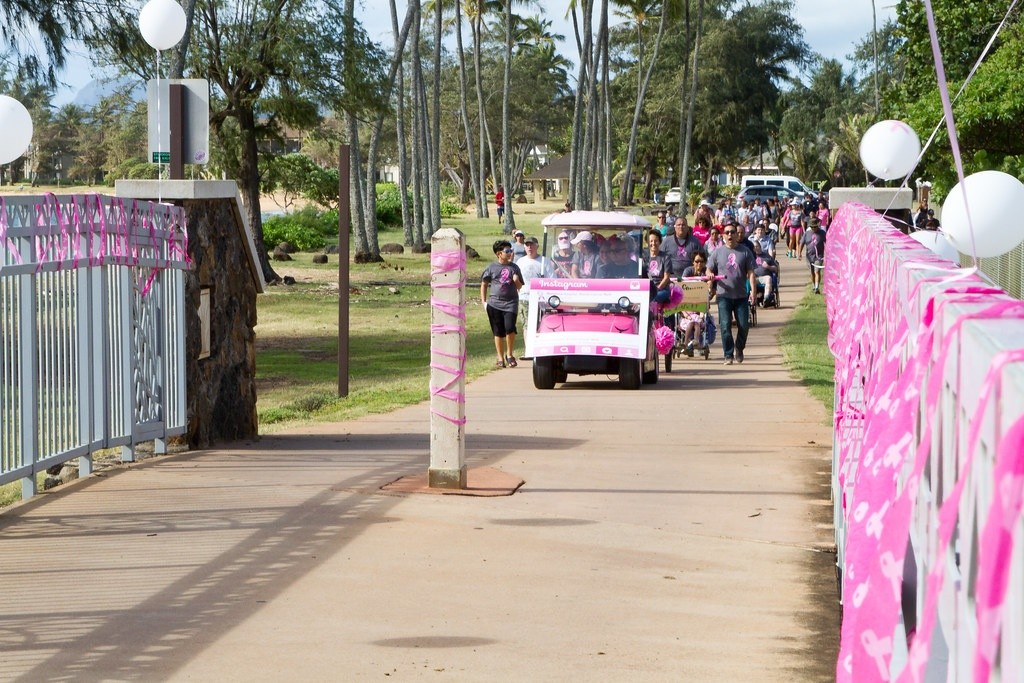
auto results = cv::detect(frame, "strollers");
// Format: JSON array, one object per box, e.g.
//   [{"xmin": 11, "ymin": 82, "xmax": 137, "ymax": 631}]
[{"xmin": 672, "ymin": 307, "xmax": 713, "ymax": 361}]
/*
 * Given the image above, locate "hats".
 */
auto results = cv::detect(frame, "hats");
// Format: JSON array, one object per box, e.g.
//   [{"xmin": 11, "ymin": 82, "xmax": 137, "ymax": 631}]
[
  {"xmin": 524, "ymin": 236, "xmax": 538, "ymax": 244},
  {"xmin": 927, "ymin": 209, "xmax": 935, "ymax": 215},
  {"xmin": 698, "ymin": 200, "xmax": 711, "ymax": 206},
  {"xmin": 789, "ymin": 200, "xmax": 800, "ymax": 206},
  {"xmin": 808, "ymin": 218, "xmax": 818, "ymax": 227},
  {"xmin": 514, "ymin": 230, "xmax": 525, "ymax": 238},
  {"xmin": 556, "ymin": 232, "xmax": 571, "ymax": 249},
  {"xmin": 569, "ymin": 231, "xmax": 592, "ymax": 245}
]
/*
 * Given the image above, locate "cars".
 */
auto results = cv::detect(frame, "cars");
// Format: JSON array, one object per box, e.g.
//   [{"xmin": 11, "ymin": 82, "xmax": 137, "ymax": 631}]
[
  {"xmin": 735, "ymin": 184, "xmax": 804, "ymax": 206},
  {"xmin": 664, "ymin": 187, "xmax": 692, "ymax": 208}
]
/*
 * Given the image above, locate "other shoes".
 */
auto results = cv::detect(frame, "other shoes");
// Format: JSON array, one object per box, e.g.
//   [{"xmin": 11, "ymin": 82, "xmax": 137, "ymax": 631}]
[
  {"xmin": 733, "ymin": 344, "xmax": 744, "ymax": 363},
  {"xmin": 786, "ymin": 251, "xmax": 798, "ymax": 258},
  {"xmin": 813, "ymin": 286, "xmax": 815, "ymax": 291},
  {"xmin": 724, "ymin": 357, "xmax": 734, "ymax": 366},
  {"xmin": 815, "ymin": 287, "xmax": 821, "ymax": 295}
]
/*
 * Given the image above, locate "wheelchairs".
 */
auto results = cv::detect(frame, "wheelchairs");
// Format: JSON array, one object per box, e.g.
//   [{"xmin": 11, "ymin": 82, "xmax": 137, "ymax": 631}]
[{"xmin": 746, "ymin": 260, "xmax": 782, "ymax": 309}]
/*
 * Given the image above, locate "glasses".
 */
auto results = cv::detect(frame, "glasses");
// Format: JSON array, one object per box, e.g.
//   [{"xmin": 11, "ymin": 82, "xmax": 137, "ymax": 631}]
[
  {"xmin": 699, "ymin": 221, "xmax": 705, "ymax": 224},
  {"xmin": 516, "ymin": 234, "xmax": 523, "ymax": 237},
  {"xmin": 809, "ymin": 227, "xmax": 816, "ymax": 228},
  {"xmin": 609, "ymin": 248, "xmax": 625, "ymax": 253},
  {"xmin": 694, "ymin": 260, "xmax": 705, "ymax": 263},
  {"xmin": 724, "ymin": 230, "xmax": 736, "ymax": 234},
  {"xmin": 558, "ymin": 236, "xmax": 568, "ymax": 240},
  {"xmin": 658, "ymin": 216, "xmax": 666, "ymax": 219},
  {"xmin": 499, "ymin": 248, "xmax": 514, "ymax": 254}
]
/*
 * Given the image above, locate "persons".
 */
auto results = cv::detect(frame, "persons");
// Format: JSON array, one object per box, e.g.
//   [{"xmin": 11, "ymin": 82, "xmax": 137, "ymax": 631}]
[
  {"xmin": 652, "ymin": 199, "xmax": 726, "ymax": 353},
  {"xmin": 481, "ymin": 240, "xmax": 526, "ymax": 368},
  {"xmin": 717, "ymin": 194, "xmax": 782, "ymax": 307},
  {"xmin": 654, "ymin": 185, "xmax": 660, "ymax": 204},
  {"xmin": 912, "ymin": 197, "xmax": 941, "ymax": 231},
  {"xmin": 550, "ymin": 228, "xmax": 641, "ymax": 279},
  {"xmin": 642, "ymin": 230, "xmax": 676, "ymax": 304},
  {"xmin": 516, "ymin": 237, "xmax": 557, "ymax": 360},
  {"xmin": 777, "ymin": 191, "xmax": 830, "ymax": 293},
  {"xmin": 495, "ymin": 186, "xmax": 505, "ymax": 224},
  {"xmin": 706, "ymin": 224, "xmax": 759, "ymax": 365},
  {"xmin": 507, "ymin": 227, "xmax": 527, "ymax": 263}
]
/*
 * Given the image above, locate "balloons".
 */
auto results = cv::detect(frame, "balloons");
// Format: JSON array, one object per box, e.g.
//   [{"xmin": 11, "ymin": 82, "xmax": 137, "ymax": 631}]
[
  {"xmin": 909, "ymin": 230, "xmax": 960, "ymax": 261},
  {"xmin": 860, "ymin": 119, "xmax": 921, "ymax": 182},
  {"xmin": 942, "ymin": 169, "xmax": 1024, "ymax": 258},
  {"xmin": 0, "ymin": 95, "xmax": 33, "ymax": 165},
  {"xmin": 139, "ymin": 0, "xmax": 187, "ymax": 51}
]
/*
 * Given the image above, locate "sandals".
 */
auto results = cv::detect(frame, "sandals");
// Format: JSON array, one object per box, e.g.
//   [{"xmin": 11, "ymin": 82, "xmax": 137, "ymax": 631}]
[
  {"xmin": 496, "ymin": 359, "xmax": 508, "ymax": 369},
  {"xmin": 504, "ymin": 355, "xmax": 517, "ymax": 368}
]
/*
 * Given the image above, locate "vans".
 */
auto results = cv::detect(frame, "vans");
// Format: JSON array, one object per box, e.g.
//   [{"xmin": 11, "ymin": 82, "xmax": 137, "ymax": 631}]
[{"xmin": 741, "ymin": 175, "xmax": 819, "ymax": 202}]
